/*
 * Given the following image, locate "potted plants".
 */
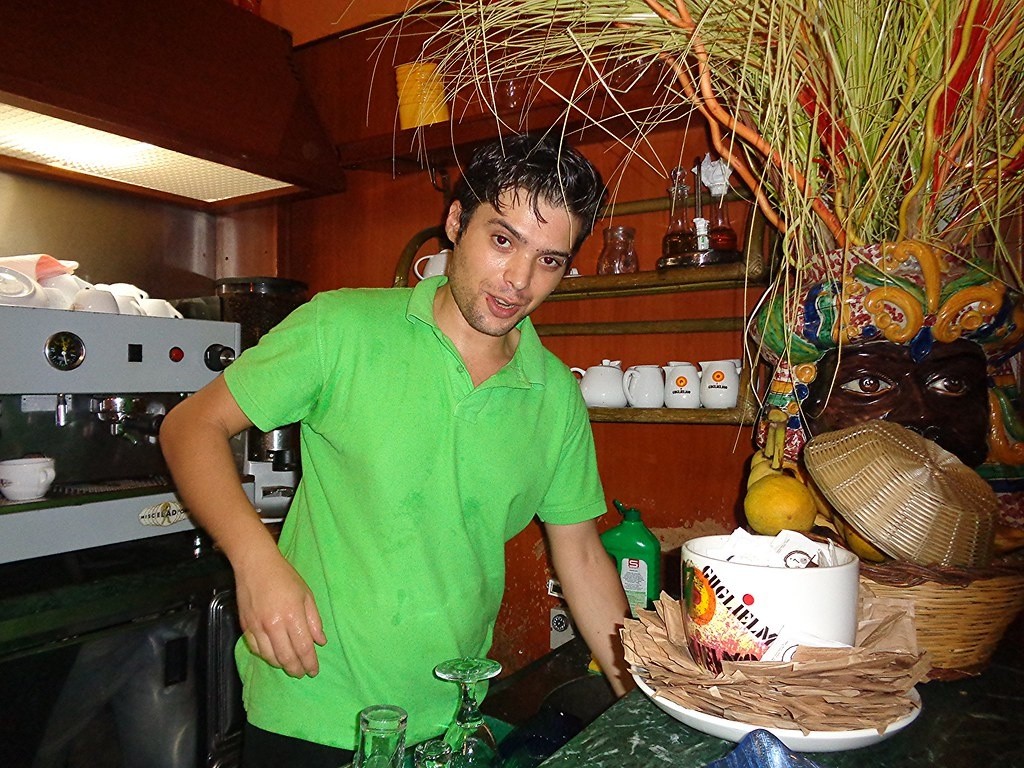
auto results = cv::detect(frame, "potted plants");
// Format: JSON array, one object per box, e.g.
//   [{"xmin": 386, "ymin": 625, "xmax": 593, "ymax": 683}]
[{"xmin": 332, "ymin": 0, "xmax": 1024, "ymax": 463}]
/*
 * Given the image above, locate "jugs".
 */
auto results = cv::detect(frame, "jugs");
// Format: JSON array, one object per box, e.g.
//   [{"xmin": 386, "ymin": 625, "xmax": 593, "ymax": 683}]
[
  {"xmin": 413, "ymin": 248, "xmax": 453, "ymax": 280},
  {"xmin": 569, "ymin": 358, "xmax": 742, "ymax": 409}
]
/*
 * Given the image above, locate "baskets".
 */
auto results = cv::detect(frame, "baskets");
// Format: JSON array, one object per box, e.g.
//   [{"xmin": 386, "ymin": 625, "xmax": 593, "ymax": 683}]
[
  {"xmin": 800, "ymin": 417, "xmax": 998, "ymax": 577},
  {"xmin": 794, "ymin": 530, "xmax": 1024, "ymax": 684}
]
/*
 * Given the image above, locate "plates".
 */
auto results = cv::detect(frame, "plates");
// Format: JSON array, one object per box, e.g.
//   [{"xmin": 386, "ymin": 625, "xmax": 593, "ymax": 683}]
[
  {"xmin": 630, "ymin": 664, "xmax": 922, "ymax": 753},
  {"xmin": 0, "ymin": 264, "xmax": 48, "ymax": 308}
]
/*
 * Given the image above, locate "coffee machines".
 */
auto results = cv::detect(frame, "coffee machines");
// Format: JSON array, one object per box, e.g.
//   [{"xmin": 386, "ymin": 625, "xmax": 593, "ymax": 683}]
[{"xmin": 214, "ymin": 276, "xmax": 310, "ymax": 472}]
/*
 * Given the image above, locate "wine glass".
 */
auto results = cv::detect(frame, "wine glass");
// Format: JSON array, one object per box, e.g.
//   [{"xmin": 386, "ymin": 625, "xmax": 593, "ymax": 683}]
[{"xmin": 433, "ymin": 656, "xmax": 503, "ymax": 768}]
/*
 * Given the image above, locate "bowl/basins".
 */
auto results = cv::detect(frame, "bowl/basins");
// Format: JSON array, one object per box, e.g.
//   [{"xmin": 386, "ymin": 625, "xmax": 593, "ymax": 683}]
[{"xmin": 680, "ymin": 534, "xmax": 860, "ymax": 678}]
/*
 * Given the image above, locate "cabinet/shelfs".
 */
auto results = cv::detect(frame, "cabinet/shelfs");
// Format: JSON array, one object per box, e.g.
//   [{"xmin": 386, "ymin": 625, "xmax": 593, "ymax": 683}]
[{"xmin": 393, "ymin": 181, "xmax": 782, "ymax": 424}]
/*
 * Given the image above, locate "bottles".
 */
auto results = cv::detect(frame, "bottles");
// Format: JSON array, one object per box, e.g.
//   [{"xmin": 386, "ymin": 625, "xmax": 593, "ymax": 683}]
[
  {"xmin": 661, "ymin": 166, "xmax": 698, "ymax": 258},
  {"xmin": 707, "ymin": 184, "xmax": 738, "ymax": 251},
  {"xmin": 597, "ymin": 224, "xmax": 638, "ymax": 274},
  {"xmin": 599, "ymin": 499, "xmax": 661, "ymax": 622},
  {"xmin": 415, "ymin": 740, "xmax": 453, "ymax": 768}
]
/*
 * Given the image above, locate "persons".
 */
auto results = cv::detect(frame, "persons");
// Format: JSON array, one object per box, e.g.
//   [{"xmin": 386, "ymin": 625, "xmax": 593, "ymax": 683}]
[
  {"xmin": 160, "ymin": 133, "xmax": 636, "ymax": 768},
  {"xmin": 750, "ymin": 241, "xmax": 1024, "ymax": 469}
]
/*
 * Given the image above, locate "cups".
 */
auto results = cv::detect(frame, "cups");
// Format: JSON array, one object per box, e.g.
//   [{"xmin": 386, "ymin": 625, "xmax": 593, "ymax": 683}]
[
  {"xmin": 39, "ymin": 273, "xmax": 184, "ymax": 319},
  {"xmin": 352, "ymin": 704, "xmax": 408, "ymax": 768},
  {"xmin": 0, "ymin": 457, "xmax": 56, "ymax": 501}
]
